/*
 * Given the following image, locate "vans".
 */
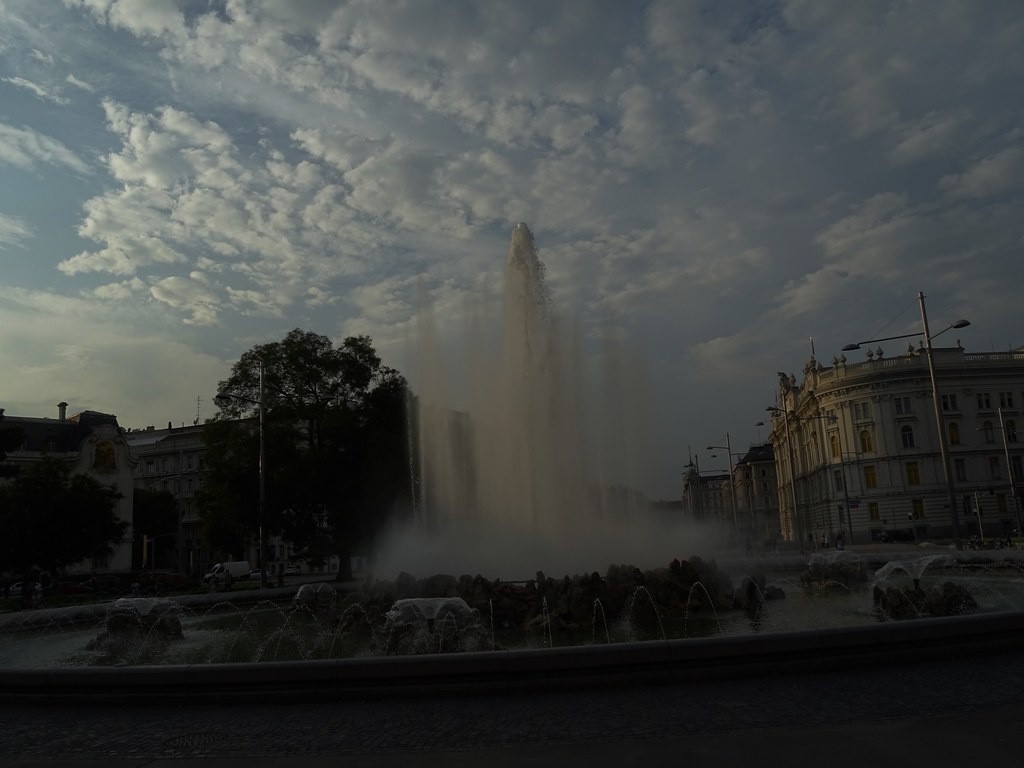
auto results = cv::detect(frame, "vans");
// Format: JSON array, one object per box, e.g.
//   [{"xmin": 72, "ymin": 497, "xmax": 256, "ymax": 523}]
[{"xmin": 204, "ymin": 560, "xmax": 251, "ymax": 586}]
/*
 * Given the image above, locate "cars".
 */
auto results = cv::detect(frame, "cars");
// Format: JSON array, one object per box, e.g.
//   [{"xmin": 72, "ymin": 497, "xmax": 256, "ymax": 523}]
[
  {"xmin": 53, "ymin": 581, "xmax": 90, "ymax": 595},
  {"xmin": 249, "ymin": 569, "xmax": 271, "ymax": 580},
  {"xmin": 0, "ymin": 582, "xmax": 43, "ymax": 594},
  {"xmin": 284, "ymin": 568, "xmax": 297, "ymax": 576},
  {"xmin": 91, "ymin": 575, "xmax": 122, "ymax": 589}
]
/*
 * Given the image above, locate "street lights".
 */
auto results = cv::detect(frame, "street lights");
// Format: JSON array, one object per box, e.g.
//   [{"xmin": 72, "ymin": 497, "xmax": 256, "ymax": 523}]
[
  {"xmin": 681, "ymin": 465, "xmax": 729, "ymax": 528},
  {"xmin": 977, "ymin": 427, "xmax": 1024, "ymax": 535},
  {"xmin": 706, "ymin": 446, "xmax": 765, "ymax": 549},
  {"xmin": 755, "ymin": 407, "xmax": 838, "ymax": 556},
  {"xmin": 215, "ymin": 391, "xmax": 337, "ymax": 600},
  {"xmin": 841, "ymin": 320, "xmax": 972, "ymax": 554},
  {"xmin": 160, "ymin": 475, "xmax": 203, "ymax": 578}
]
